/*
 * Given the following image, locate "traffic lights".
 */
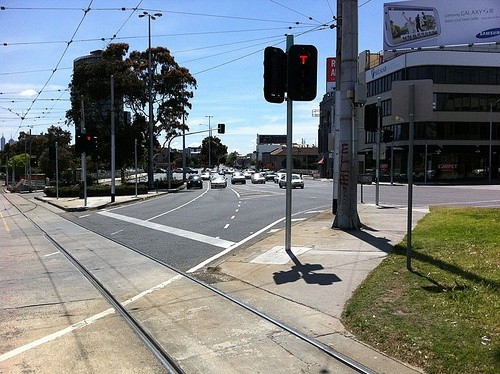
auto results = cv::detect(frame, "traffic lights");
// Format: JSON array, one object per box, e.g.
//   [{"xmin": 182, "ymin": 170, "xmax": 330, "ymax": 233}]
[
  {"xmin": 287, "ymin": 43, "xmax": 318, "ymax": 101},
  {"xmin": 84, "ymin": 133, "xmax": 92, "ymax": 152}
]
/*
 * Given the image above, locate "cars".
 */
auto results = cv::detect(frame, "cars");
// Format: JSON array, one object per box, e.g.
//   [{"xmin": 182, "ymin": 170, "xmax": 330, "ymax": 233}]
[
  {"xmin": 219, "ymin": 167, "xmax": 285, "ymax": 184},
  {"xmin": 251, "ymin": 173, "xmax": 265, "ymax": 183},
  {"xmin": 279, "ymin": 174, "xmax": 304, "ymax": 189},
  {"xmin": 210, "ymin": 175, "xmax": 228, "ymax": 188},
  {"xmin": 155, "ymin": 168, "xmax": 210, "ymax": 179}
]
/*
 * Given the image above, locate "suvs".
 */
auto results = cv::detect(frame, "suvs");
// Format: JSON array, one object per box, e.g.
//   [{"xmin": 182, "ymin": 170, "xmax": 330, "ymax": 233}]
[
  {"xmin": 231, "ymin": 172, "xmax": 246, "ymax": 184},
  {"xmin": 186, "ymin": 175, "xmax": 203, "ymax": 189}
]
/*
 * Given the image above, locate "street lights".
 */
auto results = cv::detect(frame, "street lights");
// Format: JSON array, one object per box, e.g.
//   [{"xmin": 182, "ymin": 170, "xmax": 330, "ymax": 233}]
[
  {"xmin": 199, "ymin": 124, "xmax": 212, "ymax": 136},
  {"xmin": 204, "ymin": 115, "xmax": 213, "ymax": 167}
]
